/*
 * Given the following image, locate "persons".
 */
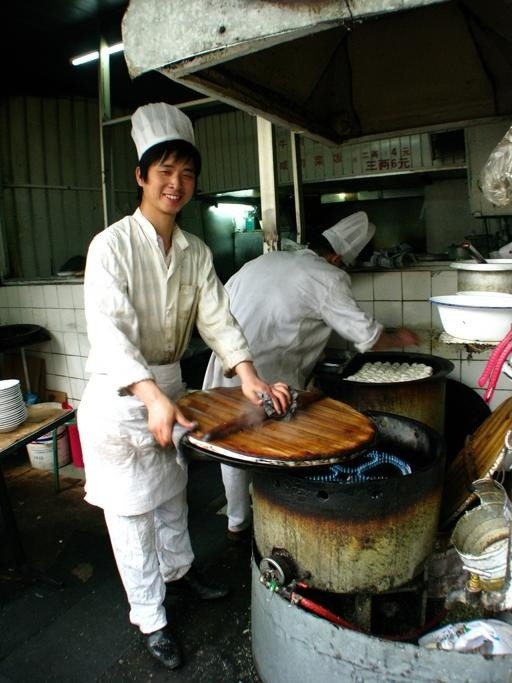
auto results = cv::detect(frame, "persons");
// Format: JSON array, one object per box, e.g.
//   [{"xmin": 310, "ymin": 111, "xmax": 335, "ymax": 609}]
[
  {"xmin": 76, "ymin": 139, "xmax": 292, "ymax": 670},
  {"xmin": 201, "ymin": 232, "xmax": 423, "ymax": 535}
]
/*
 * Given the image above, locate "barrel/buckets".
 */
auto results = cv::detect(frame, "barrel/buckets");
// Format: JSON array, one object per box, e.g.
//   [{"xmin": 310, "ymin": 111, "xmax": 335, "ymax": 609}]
[
  {"xmin": 448, "ymin": 477, "xmax": 511, "ymax": 592},
  {"xmin": 26, "ymin": 425, "xmax": 71, "ymax": 471}
]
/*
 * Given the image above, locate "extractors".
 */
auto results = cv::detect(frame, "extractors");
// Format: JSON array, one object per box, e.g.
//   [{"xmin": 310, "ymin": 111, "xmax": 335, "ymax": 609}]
[
  {"xmin": 320, "ymin": 183, "xmax": 426, "ymax": 207},
  {"xmin": 121, "ymin": 0, "xmax": 512, "ymax": 154}
]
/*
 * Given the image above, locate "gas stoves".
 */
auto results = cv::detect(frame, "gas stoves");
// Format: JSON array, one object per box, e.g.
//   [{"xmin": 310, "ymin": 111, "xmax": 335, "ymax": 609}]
[{"xmin": 294, "ymin": 445, "xmax": 414, "ymax": 484}]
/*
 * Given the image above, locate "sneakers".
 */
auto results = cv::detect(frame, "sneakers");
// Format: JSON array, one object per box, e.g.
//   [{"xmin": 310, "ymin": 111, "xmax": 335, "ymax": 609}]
[{"xmin": 225, "ymin": 529, "xmax": 251, "ymax": 545}]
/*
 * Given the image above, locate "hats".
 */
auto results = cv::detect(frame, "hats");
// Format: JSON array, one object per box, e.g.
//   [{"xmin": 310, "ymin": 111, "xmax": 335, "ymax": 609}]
[
  {"xmin": 131, "ymin": 102, "xmax": 195, "ymax": 162},
  {"xmin": 322, "ymin": 212, "xmax": 376, "ymax": 266}
]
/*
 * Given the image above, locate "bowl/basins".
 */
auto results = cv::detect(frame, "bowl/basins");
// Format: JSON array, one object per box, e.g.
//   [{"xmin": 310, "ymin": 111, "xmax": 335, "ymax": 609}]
[{"xmin": 428, "ymin": 290, "xmax": 512, "ymax": 342}]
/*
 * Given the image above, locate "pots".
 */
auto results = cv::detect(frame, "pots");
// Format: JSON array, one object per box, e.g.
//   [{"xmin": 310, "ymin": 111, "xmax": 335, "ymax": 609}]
[
  {"xmin": 178, "ymin": 429, "xmax": 381, "ymax": 476},
  {"xmin": 448, "ymin": 258, "xmax": 512, "ymax": 293},
  {"xmin": 337, "ymin": 351, "xmax": 455, "ymax": 389},
  {"xmin": 446, "ymin": 245, "xmax": 470, "ymax": 261}
]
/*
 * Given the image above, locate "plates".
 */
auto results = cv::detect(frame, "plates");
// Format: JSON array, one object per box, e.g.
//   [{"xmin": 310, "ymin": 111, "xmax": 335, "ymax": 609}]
[
  {"xmin": 56, "ymin": 271, "xmax": 84, "ymax": 277},
  {"xmin": 0, "ymin": 378, "xmax": 29, "ymax": 436}
]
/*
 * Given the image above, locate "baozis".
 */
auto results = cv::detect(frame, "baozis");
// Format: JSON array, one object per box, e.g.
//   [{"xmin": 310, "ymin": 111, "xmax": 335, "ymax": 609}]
[{"xmin": 343, "ymin": 361, "xmax": 433, "ymax": 383}]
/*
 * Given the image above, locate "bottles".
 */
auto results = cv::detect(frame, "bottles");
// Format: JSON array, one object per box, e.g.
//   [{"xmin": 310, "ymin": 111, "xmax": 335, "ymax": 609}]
[{"xmin": 433, "ymin": 152, "xmax": 465, "ymax": 165}]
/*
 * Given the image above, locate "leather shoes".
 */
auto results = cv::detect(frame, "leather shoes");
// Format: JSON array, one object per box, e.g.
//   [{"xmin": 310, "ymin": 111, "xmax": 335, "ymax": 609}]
[
  {"xmin": 166, "ymin": 569, "xmax": 229, "ymax": 600},
  {"xmin": 148, "ymin": 630, "xmax": 181, "ymax": 669}
]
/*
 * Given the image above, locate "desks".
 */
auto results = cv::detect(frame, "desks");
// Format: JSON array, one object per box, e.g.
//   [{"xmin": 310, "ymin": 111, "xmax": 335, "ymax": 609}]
[{"xmin": 0, "ymin": 404, "xmax": 74, "ymax": 557}]
